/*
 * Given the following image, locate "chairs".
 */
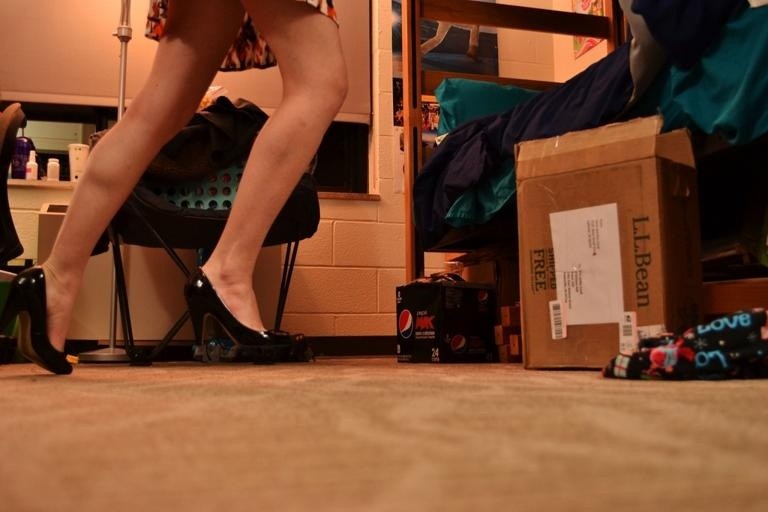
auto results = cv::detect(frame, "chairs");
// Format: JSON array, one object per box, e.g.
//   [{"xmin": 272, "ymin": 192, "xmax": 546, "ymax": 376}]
[{"xmin": 88, "ymin": 110, "xmax": 318, "ymax": 367}]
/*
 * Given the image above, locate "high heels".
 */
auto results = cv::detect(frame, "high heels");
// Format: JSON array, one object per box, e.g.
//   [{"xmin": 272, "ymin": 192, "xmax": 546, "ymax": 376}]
[
  {"xmin": 0, "ymin": 266, "xmax": 74, "ymax": 375},
  {"xmin": 184, "ymin": 266, "xmax": 306, "ymax": 363}
]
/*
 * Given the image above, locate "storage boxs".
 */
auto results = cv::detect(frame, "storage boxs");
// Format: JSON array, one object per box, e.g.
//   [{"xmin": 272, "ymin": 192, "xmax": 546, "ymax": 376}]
[{"xmin": 513, "ymin": 116, "xmax": 704, "ymax": 374}]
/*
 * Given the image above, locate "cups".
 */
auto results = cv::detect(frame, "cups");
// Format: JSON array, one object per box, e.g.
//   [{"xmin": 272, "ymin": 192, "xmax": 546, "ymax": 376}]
[{"xmin": 69, "ymin": 144, "xmax": 90, "ymax": 182}]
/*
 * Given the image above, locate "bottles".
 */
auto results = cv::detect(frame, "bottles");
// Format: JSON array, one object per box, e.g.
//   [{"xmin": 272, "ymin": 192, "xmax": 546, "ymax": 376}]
[
  {"xmin": 46, "ymin": 158, "xmax": 59, "ymax": 180},
  {"xmin": 25, "ymin": 150, "xmax": 39, "ymax": 181}
]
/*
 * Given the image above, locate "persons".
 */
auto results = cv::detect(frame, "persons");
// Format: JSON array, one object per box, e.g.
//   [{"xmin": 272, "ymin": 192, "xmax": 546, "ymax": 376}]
[{"xmin": 0, "ymin": 0, "xmax": 350, "ymax": 377}]
